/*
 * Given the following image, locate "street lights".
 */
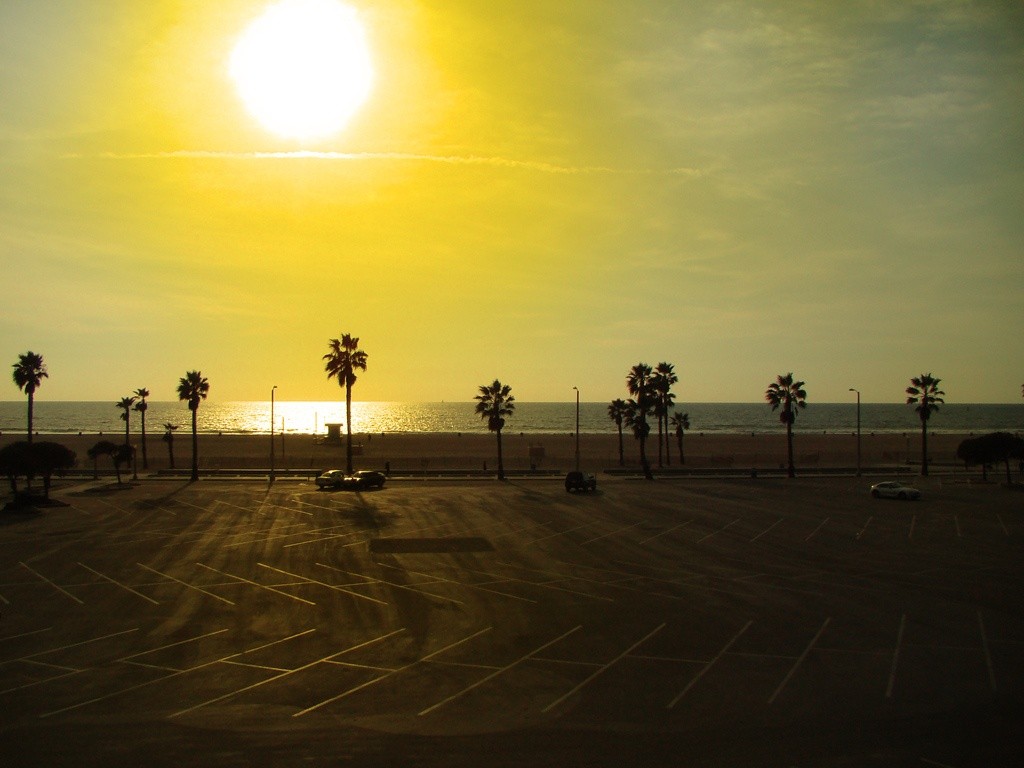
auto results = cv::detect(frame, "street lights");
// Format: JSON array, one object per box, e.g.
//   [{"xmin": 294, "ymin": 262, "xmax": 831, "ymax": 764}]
[
  {"xmin": 271, "ymin": 385, "xmax": 278, "ymax": 481},
  {"xmin": 849, "ymin": 388, "xmax": 861, "ymax": 477},
  {"xmin": 573, "ymin": 387, "xmax": 580, "ymax": 469}
]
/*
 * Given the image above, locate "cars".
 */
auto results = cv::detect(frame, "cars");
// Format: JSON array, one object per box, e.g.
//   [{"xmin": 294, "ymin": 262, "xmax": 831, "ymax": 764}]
[
  {"xmin": 315, "ymin": 469, "xmax": 354, "ymax": 490},
  {"xmin": 341, "ymin": 470, "xmax": 386, "ymax": 490},
  {"xmin": 564, "ymin": 469, "xmax": 598, "ymax": 492},
  {"xmin": 869, "ymin": 480, "xmax": 921, "ymax": 501}
]
